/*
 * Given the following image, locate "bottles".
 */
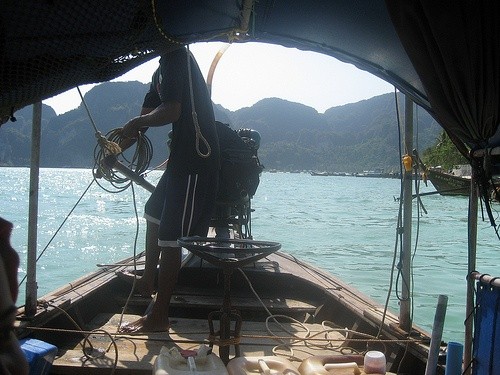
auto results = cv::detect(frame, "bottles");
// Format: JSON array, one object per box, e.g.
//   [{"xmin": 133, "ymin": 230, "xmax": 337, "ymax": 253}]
[{"xmin": 363, "ymin": 351, "xmax": 386, "ymax": 375}]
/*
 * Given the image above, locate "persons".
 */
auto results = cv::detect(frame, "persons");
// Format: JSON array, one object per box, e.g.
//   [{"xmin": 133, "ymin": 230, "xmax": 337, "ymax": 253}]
[{"xmin": 90, "ymin": 39, "xmax": 223, "ymax": 342}]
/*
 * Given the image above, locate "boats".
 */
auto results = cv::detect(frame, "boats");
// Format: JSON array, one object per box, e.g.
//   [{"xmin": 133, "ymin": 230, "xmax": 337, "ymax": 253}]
[
  {"xmin": 268, "ymin": 168, "xmax": 423, "ymax": 181},
  {"xmin": 426, "ymin": 169, "xmax": 500, "ymax": 201}
]
